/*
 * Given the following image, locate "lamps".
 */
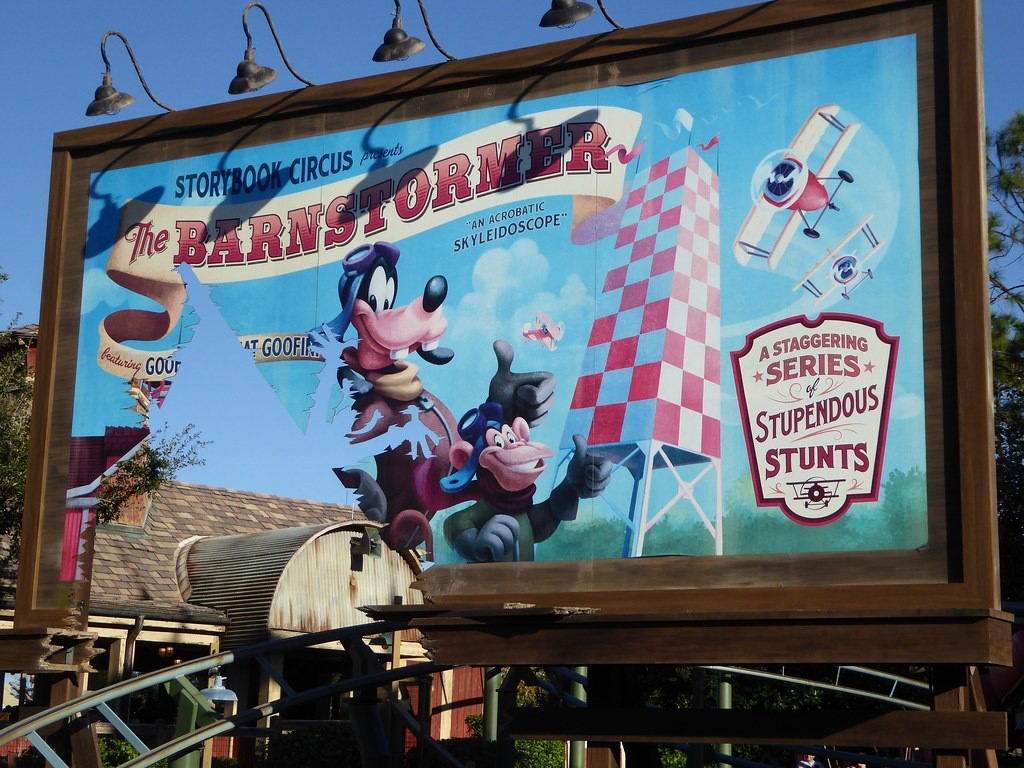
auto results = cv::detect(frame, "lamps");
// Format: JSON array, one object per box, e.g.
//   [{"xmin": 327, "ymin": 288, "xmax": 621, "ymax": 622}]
[
  {"xmin": 372, "ymin": 0, "xmax": 454, "ymax": 62},
  {"xmin": 539, "ymin": 0, "xmax": 621, "ymax": 29},
  {"xmin": 228, "ymin": 3, "xmax": 315, "ymax": 95},
  {"xmin": 86, "ymin": 31, "xmax": 176, "ymax": 116}
]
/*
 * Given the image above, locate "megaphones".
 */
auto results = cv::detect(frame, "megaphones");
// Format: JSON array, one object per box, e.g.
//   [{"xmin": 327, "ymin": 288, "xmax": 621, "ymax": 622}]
[{"xmin": 353, "ymin": 526, "xmax": 382, "ymax": 558}]
